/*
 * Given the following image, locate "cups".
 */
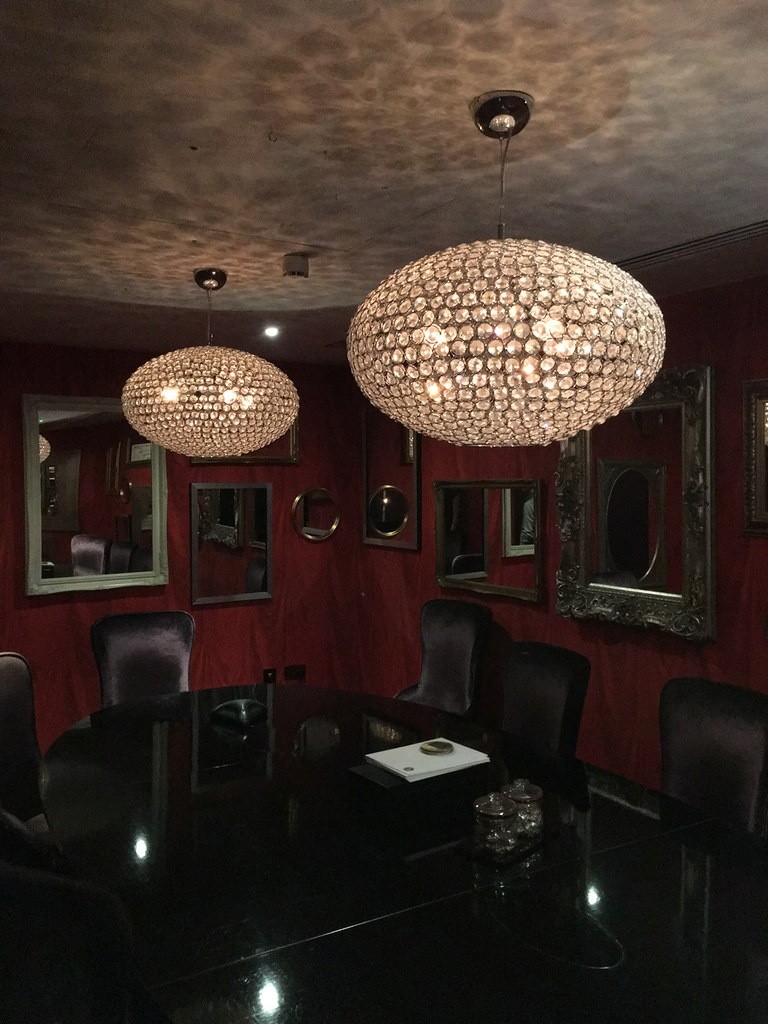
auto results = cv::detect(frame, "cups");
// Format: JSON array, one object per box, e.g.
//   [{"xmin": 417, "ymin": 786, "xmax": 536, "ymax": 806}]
[
  {"xmin": 473, "ymin": 791, "xmax": 517, "ymax": 853},
  {"xmin": 500, "ymin": 778, "xmax": 543, "ymax": 839}
]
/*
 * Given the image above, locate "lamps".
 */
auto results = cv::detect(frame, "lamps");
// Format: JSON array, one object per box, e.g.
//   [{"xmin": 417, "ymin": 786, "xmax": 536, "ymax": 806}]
[
  {"xmin": 120, "ymin": 267, "xmax": 301, "ymax": 459},
  {"xmin": 38, "ymin": 433, "xmax": 52, "ymax": 464},
  {"xmin": 344, "ymin": 89, "xmax": 668, "ymax": 449}
]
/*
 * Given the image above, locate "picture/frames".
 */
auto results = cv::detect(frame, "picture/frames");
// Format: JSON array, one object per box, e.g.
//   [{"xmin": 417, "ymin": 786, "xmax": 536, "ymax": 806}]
[
  {"xmin": 442, "ymin": 487, "xmax": 493, "ymax": 583},
  {"xmin": 501, "ymin": 485, "xmax": 540, "ymax": 560},
  {"xmin": 550, "ymin": 361, "xmax": 720, "ymax": 649},
  {"xmin": 432, "ymin": 477, "xmax": 547, "ymax": 606}
]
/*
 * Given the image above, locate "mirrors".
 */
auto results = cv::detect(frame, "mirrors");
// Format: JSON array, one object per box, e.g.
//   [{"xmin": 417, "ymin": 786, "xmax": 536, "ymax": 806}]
[
  {"xmin": 594, "ymin": 456, "xmax": 668, "ymax": 589},
  {"xmin": 741, "ymin": 374, "xmax": 768, "ymax": 538},
  {"xmin": 189, "ymin": 481, "xmax": 273, "ymax": 606},
  {"xmin": 290, "ymin": 486, "xmax": 342, "ymax": 543},
  {"xmin": 362, "ymin": 398, "xmax": 419, "ymax": 553},
  {"xmin": 20, "ymin": 391, "xmax": 171, "ymax": 597},
  {"xmin": 191, "ymin": 417, "xmax": 303, "ymax": 464},
  {"xmin": 367, "ymin": 484, "xmax": 411, "ymax": 536}
]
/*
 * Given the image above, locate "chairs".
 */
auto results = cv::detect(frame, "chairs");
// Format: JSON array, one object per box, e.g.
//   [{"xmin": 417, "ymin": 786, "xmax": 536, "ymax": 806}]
[
  {"xmin": 0, "ymin": 598, "xmax": 768, "ymax": 1024},
  {"xmin": 68, "ymin": 534, "xmax": 154, "ymax": 576}
]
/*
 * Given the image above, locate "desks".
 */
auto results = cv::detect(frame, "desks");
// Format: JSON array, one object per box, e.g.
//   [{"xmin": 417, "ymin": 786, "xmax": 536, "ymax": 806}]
[{"xmin": 43, "ymin": 682, "xmax": 768, "ymax": 1024}]
